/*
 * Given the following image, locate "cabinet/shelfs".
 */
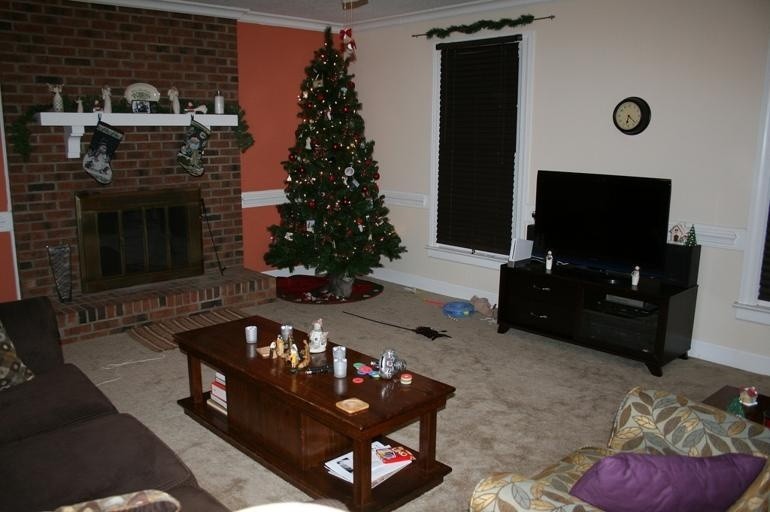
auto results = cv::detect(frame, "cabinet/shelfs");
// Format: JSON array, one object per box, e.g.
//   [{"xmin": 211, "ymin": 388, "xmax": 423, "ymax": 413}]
[{"xmin": 495, "ymin": 258, "xmax": 698, "ymax": 377}]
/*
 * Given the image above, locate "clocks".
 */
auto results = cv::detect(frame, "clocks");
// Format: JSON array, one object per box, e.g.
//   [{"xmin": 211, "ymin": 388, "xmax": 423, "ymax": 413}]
[{"xmin": 613, "ymin": 97, "xmax": 650, "ymax": 135}]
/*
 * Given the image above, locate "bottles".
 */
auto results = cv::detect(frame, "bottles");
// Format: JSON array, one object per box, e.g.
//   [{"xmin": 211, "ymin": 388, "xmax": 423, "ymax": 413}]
[
  {"xmin": 51, "ymin": 81, "xmax": 67, "ymax": 112},
  {"xmin": 214, "ymin": 90, "xmax": 225, "ymax": 114},
  {"xmin": 545, "ymin": 249, "xmax": 553, "ymax": 271},
  {"xmin": 632, "ymin": 265, "xmax": 641, "ymax": 287}
]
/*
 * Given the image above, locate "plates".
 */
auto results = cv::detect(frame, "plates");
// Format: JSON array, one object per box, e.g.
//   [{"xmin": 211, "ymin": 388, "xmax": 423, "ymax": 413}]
[{"xmin": 125, "ymin": 82, "xmax": 161, "ymax": 104}]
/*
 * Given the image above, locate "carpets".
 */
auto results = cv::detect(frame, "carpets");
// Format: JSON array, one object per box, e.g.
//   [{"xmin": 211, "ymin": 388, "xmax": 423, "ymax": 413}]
[{"xmin": 127, "ymin": 308, "xmax": 249, "ymax": 354}]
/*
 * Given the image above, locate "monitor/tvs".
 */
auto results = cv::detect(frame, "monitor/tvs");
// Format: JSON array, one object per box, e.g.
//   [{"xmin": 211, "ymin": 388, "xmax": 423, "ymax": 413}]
[{"xmin": 530, "ymin": 170, "xmax": 672, "ymax": 284}]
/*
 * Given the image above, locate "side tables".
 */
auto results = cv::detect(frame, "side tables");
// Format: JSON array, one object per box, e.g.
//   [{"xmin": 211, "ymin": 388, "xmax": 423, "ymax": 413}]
[{"xmin": 702, "ymin": 385, "xmax": 770, "ymax": 426}]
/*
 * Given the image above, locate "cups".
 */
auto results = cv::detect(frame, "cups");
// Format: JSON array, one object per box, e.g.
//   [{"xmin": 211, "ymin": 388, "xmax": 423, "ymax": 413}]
[
  {"xmin": 280, "ymin": 325, "xmax": 293, "ymax": 343},
  {"xmin": 245, "ymin": 325, "xmax": 258, "ymax": 343},
  {"xmin": 333, "ymin": 346, "xmax": 348, "ymax": 359},
  {"xmin": 333, "ymin": 359, "xmax": 347, "ymax": 378}
]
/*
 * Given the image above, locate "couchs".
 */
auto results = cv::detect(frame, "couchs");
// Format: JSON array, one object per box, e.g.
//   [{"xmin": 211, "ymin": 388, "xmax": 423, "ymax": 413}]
[
  {"xmin": 471, "ymin": 385, "xmax": 770, "ymax": 512},
  {"xmin": 0, "ymin": 297, "xmax": 231, "ymax": 512}
]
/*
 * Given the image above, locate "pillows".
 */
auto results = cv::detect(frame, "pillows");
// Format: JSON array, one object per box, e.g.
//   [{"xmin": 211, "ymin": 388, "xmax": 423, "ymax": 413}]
[
  {"xmin": 0, "ymin": 318, "xmax": 35, "ymax": 390},
  {"xmin": 569, "ymin": 452, "xmax": 765, "ymax": 512},
  {"xmin": 55, "ymin": 491, "xmax": 180, "ymax": 512}
]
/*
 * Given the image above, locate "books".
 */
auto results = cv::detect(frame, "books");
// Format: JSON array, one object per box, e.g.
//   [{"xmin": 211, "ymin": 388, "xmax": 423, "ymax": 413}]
[
  {"xmin": 207, "ymin": 372, "xmax": 228, "ymax": 416},
  {"xmin": 323, "ymin": 441, "xmax": 416, "ymax": 489}
]
